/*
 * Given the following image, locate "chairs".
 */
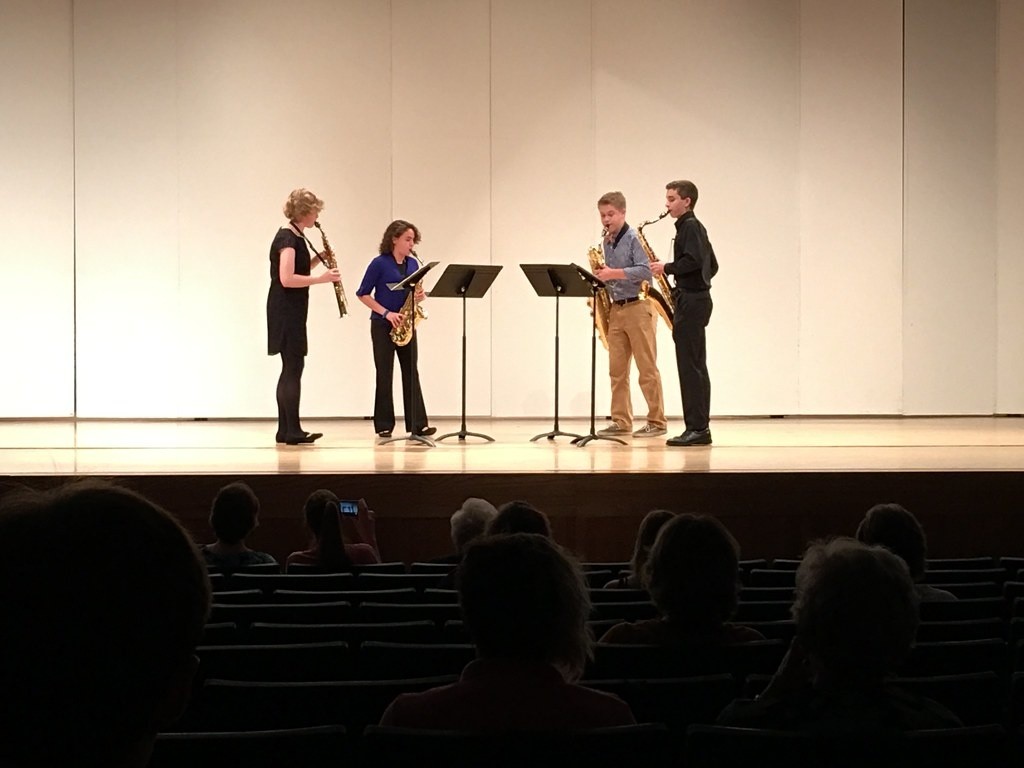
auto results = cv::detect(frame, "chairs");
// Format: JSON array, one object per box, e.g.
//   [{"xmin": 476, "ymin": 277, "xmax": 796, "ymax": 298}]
[{"xmin": 153, "ymin": 546, "xmax": 1024, "ymax": 768}]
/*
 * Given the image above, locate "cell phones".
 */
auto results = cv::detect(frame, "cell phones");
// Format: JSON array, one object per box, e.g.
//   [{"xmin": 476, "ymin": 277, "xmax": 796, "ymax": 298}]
[{"xmin": 339, "ymin": 500, "xmax": 359, "ymax": 516}]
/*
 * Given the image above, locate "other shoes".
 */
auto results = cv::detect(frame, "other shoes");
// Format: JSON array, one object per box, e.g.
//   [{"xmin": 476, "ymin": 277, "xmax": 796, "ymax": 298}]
[
  {"xmin": 275, "ymin": 433, "xmax": 315, "ymax": 443},
  {"xmin": 412, "ymin": 426, "xmax": 436, "ymax": 436},
  {"xmin": 380, "ymin": 430, "xmax": 391, "ymax": 437},
  {"xmin": 286, "ymin": 431, "xmax": 323, "ymax": 445}
]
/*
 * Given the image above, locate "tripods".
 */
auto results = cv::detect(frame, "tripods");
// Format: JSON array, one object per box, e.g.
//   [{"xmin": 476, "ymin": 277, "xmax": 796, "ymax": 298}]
[
  {"xmin": 533, "ymin": 263, "xmax": 628, "ymax": 442},
  {"xmin": 378, "ymin": 261, "xmax": 503, "ymax": 447}
]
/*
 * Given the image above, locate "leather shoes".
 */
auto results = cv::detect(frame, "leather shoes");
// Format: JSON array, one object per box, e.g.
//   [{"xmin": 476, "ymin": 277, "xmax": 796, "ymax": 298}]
[{"xmin": 667, "ymin": 428, "xmax": 712, "ymax": 445}]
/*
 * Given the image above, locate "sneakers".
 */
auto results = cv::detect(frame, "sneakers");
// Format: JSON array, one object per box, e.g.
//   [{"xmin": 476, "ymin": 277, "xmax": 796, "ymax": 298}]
[
  {"xmin": 632, "ymin": 422, "xmax": 668, "ymax": 436},
  {"xmin": 597, "ymin": 423, "xmax": 633, "ymax": 436}
]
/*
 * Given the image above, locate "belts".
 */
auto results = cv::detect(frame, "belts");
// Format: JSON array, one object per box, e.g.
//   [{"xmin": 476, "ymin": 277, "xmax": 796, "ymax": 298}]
[{"xmin": 614, "ymin": 296, "xmax": 639, "ymax": 306}]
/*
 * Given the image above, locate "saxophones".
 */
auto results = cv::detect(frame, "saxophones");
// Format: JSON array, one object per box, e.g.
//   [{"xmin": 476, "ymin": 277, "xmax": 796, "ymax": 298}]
[
  {"xmin": 313, "ymin": 220, "xmax": 350, "ymax": 318},
  {"xmin": 586, "ymin": 224, "xmax": 610, "ymax": 349},
  {"xmin": 388, "ymin": 248, "xmax": 429, "ymax": 346},
  {"xmin": 636, "ymin": 210, "xmax": 674, "ymax": 331}
]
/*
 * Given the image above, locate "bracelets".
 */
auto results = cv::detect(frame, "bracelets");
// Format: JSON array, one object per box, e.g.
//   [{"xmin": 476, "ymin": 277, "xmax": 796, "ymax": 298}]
[{"xmin": 382, "ymin": 309, "xmax": 389, "ymax": 319}]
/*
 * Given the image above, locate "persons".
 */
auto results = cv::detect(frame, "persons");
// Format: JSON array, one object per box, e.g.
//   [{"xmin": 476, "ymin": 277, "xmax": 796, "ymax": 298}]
[
  {"xmin": 650, "ymin": 180, "xmax": 719, "ymax": 446},
  {"xmin": 597, "ymin": 190, "xmax": 668, "ymax": 436},
  {"xmin": 0, "ymin": 477, "xmax": 213, "ymax": 768},
  {"xmin": 267, "ymin": 188, "xmax": 342, "ymax": 443},
  {"xmin": 417, "ymin": 498, "xmax": 499, "ymax": 563},
  {"xmin": 443, "ymin": 500, "xmax": 551, "ymax": 590},
  {"xmin": 716, "ymin": 534, "xmax": 964, "ymax": 729},
  {"xmin": 285, "ymin": 489, "xmax": 391, "ymax": 587},
  {"xmin": 197, "ymin": 479, "xmax": 276, "ymax": 565},
  {"xmin": 856, "ymin": 502, "xmax": 956, "ymax": 602},
  {"xmin": 381, "ymin": 533, "xmax": 637, "ymax": 729},
  {"xmin": 355, "ymin": 219, "xmax": 437, "ymax": 436},
  {"xmin": 597, "ymin": 511, "xmax": 767, "ymax": 642},
  {"xmin": 602, "ymin": 510, "xmax": 677, "ymax": 588}
]
